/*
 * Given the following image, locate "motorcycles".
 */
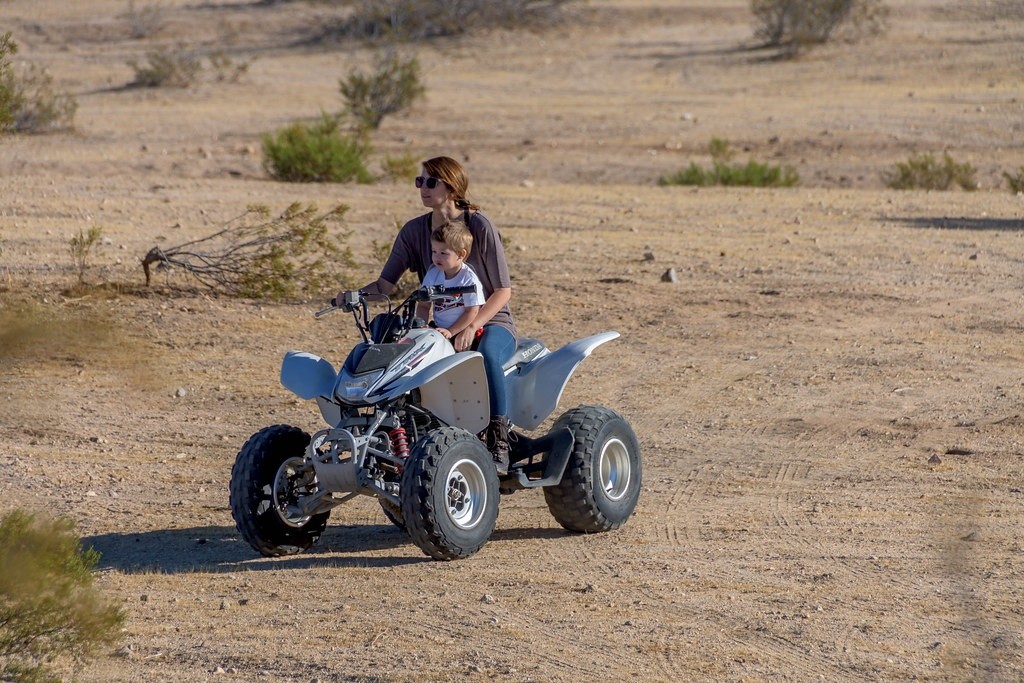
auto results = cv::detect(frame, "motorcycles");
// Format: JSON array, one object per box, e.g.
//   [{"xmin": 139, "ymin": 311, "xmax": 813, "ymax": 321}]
[{"xmin": 227, "ymin": 284, "xmax": 643, "ymax": 562}]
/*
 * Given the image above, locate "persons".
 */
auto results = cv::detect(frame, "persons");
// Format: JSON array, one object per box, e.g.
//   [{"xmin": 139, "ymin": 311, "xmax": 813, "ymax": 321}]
[
  {"xmin": 333, "ymin": 155, "xmax": 517, "ymax": 477},
  {"xmin": 413, "ymin": 220, "xmax": 487, "ymax": 343}
]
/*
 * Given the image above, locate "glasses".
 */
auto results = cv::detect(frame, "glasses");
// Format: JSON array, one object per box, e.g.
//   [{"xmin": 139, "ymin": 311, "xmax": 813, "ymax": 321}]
[{"xmin": 415, "ymin": 176, "xmax": 437, "ymax": 189}]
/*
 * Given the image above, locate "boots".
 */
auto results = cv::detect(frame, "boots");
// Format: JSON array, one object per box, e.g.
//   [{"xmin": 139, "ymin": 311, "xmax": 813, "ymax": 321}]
[{"xmin": 486, "ymin": 414, "xmax": 519, "ymax": 474}]
